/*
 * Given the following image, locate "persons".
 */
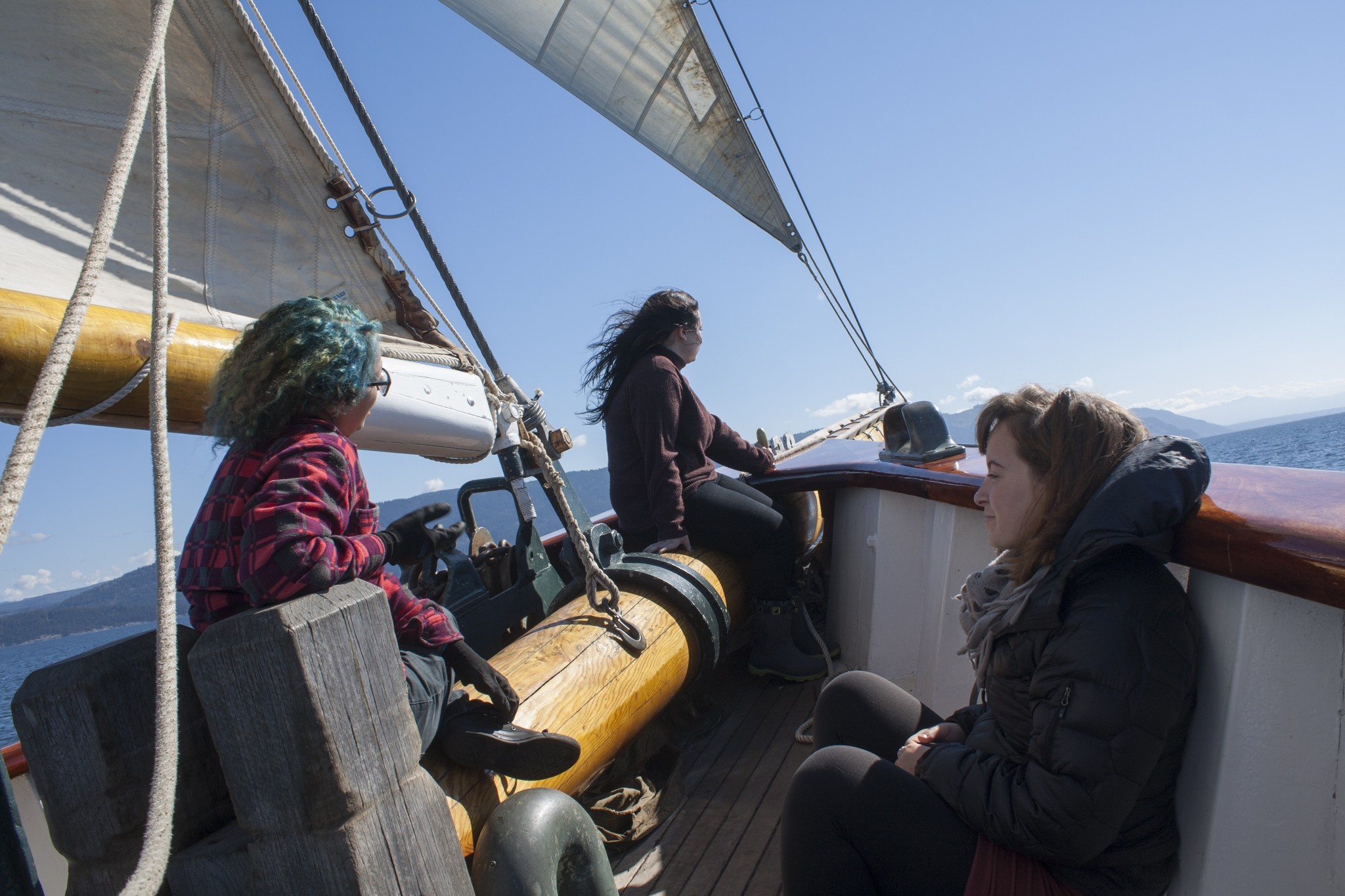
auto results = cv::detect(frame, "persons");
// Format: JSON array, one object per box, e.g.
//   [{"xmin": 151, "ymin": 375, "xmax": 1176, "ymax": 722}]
[
  {"xmin": 572, "ymin": 289, "xmax": 840, "ymax": 683},
  {"xmin": 777, "ymin": 384, "xmax": 1215, "ymax": 896},
  {"xmin": 178, "ymin": 294, "xmax": 578, "ymax": 780}
]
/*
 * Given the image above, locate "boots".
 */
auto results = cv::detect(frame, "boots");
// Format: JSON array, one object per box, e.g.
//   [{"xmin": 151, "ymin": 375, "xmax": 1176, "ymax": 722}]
[{"xmin": 749, "ymin": 594, "xmax": 841, "ymax": 681}]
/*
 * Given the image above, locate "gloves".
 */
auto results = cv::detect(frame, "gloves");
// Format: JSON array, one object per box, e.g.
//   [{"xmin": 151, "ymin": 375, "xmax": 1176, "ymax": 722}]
[
  {"xmin": 442, "ymin": 639, "xmax": 519, "ymax": 725},
  {"xmin": 370, "ymin": 502, "xmax": 466, "ymax": 567}
]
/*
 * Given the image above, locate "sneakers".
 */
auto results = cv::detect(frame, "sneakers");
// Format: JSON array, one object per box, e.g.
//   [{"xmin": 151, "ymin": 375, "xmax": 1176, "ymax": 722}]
[{"xmin": 443, "ymin": 712, "xmax": 581, "ymax": 781}]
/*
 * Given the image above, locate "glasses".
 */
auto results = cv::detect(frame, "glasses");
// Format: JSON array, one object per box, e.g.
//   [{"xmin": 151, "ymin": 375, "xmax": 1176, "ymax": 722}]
[{"xmin": 360, "ymin": 367, "xmax": 392, "ymax": 397}]
